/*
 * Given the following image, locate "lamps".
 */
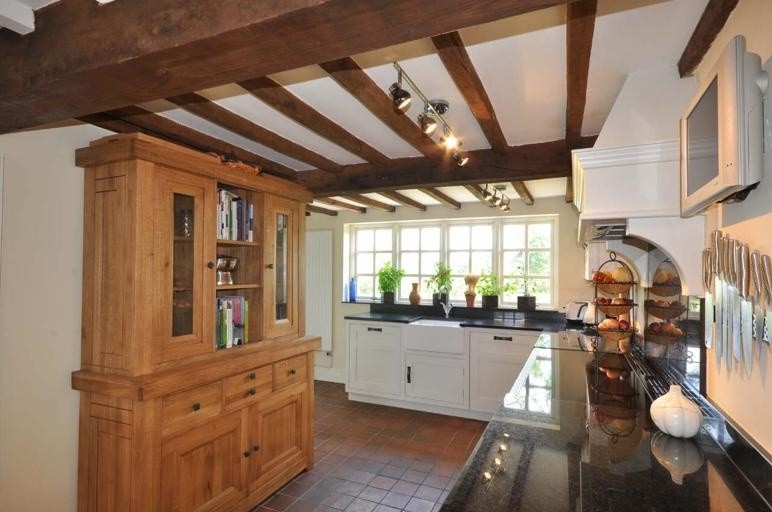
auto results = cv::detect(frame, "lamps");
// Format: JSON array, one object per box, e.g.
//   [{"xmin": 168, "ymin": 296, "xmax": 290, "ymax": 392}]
[{"xmin": 390, "ymin": 70, "xmax": 472, "ymax": 169}]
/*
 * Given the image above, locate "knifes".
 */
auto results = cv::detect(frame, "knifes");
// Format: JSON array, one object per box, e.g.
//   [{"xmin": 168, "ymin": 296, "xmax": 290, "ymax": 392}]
[{"xmin": 703, "ymin": 230, "xmax": 772, "ymax": 390}]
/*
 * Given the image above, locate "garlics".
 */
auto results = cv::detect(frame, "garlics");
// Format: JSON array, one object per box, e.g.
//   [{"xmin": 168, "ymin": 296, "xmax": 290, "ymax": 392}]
[
  {"xmin": 650, "ymin": 431, "xmax": 704, "ymax": 486},
  {"xmin": 649, "ymin": 385, "xmax": 703, "ymax": 438}
]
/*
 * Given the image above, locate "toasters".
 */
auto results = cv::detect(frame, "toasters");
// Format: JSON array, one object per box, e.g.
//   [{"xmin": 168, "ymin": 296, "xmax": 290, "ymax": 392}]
[{"xmin": 567, "ymin": 301, "xmax": 588, "ymax": 320}]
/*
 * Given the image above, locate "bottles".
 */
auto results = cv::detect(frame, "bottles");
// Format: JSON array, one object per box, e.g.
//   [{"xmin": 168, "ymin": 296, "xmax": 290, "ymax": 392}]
[
  {"xmin": 350, "ymin": 277, "xmax": 355, "ymax": 301},
  {"xmin": 410, "ymin": 284, "xmax": 421, "ymax": 303},
  {"xmin": 176, "ymin": 209, "xmax": 193, "ymax": 237}
]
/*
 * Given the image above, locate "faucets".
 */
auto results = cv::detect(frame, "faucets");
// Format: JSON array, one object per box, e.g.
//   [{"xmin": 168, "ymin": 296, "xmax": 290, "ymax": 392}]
[{"xmin": 441, "ymin": 302, "xmax": 452, "ymax": 318}]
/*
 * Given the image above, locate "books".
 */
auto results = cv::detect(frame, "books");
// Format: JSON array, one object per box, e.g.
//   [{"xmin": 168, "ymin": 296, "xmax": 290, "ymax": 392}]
[
  {"xmin": 217, "ymin": 188, "xmax": 254, "ymax": 242},
  {"xmin": 215, "ymin": 295, "xmax": 249, "ymax": 350}
]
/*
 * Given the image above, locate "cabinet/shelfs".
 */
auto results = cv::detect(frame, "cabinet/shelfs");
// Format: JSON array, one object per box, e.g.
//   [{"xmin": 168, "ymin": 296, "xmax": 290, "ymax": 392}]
[
  {"xmin": 75, "ymin": 131, "xmax": 322, "ymax": 511},
  {"xmin": 403, "ymin": 351, "xmax": 465, "ymax": 404},
  {"xmin": 644, "ymin": 283, "xmax": 689, "ymax": 352},
  {"xmin": 465, "ymin": 327, "xmax": 554, "ymax": 423},
  {"xmin": 345, "ymin": 319, "xmax": 402, "ymax": 397},
  {"xmin": 591, "ymin": 281, "xmax": 637, "ymax": 353}
]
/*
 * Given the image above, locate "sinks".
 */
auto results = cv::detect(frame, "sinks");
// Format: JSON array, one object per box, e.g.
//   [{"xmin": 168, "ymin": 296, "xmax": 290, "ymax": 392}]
[{"xmin": 411, "ymin": 319, "xmax": 462, "ymax": 327}]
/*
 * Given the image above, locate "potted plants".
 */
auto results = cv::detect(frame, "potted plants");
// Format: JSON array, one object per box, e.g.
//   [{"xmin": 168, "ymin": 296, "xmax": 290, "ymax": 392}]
[
  {"xmin": 514, "ymin": 263, "xmax": 539, "ymax": 311},
  {"xmin": 476, "ymin": 267, "xmax": 515, "ymax": 307},
  {"xmin": 377, "ymin": 259, "xmax": 406, "ymax": 303},
  {"xmin": 424, "ymin": 260, "xmax": 455, "ymax": 306}
]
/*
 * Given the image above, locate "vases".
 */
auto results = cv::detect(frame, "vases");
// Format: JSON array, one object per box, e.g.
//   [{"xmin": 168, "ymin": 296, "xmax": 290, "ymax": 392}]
[{"xmin": 409, "ymin": 282, "xmax": 421, "ymax": 304}]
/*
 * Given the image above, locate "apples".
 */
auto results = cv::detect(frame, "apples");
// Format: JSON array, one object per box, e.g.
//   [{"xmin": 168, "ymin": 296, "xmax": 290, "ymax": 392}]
[
  {"xmin": 655, "ymin": 270, "xmax": 680, "ymax": 284},
  {"xmin": 646, "ymin": 299, "xmax": 682, "ymax": 308},
  {"xmin": 593, "ymin": 297, "xmax": 635, "ymax": 305},
  {"xmin": 592, "ymin": 266, "xmax": 631, "ymax": 283},
  {"xmin": 598, "ymin": 318, "xmax": 629, "ymax": 331},
  {"xmin": 649, "ymin": 321, "xmax": 684, "ymax": 336},
  {"xmin": 594, "ymin": 414, "xmax": 633, "ymax": 430},
  {"xmin": 599, "ymin": 368, "xmax": 628, "ymax": 380}
]
